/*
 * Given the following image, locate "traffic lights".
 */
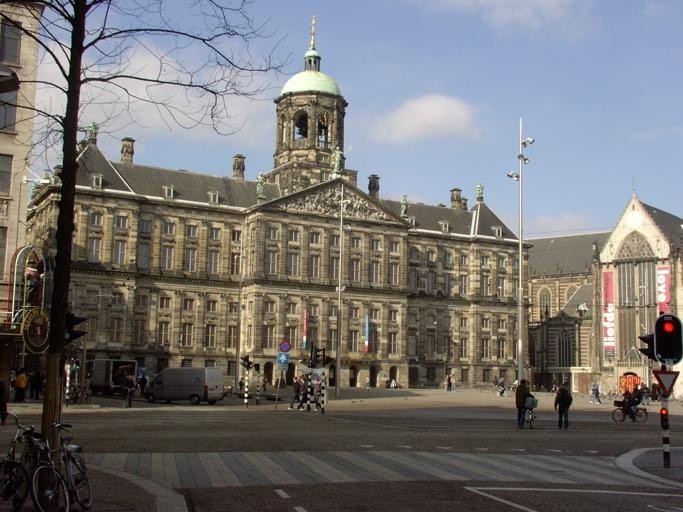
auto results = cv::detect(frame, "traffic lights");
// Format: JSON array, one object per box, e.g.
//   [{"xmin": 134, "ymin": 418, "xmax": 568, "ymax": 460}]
[
  {"xmin": 239, "ymin": 356, "xmax": 250, "ymax": 369},
  {"xmin": 325, "ymin": 357, "xmax": 335, "ymax": 366},
  {"xmin": 653, "ymin": 313, "xmax": 683, "ymax": 366},
  {"xmin": 65, "ymin": 310, "xmax": 91, "ymax": 347},
  {"xmin": 635, "ymin": 332, "xmax": 660, "ymax": 365},
  {"xmin": 660, "ymin": 407, "xmax": 670, "ymax": 430},
  {"xmin": 313, "ymin": 346, "xmax": 324, "ymax": 365},
  {"xmin": 249, "ymin": 361, "xmax": 256, "ymax": 370}
]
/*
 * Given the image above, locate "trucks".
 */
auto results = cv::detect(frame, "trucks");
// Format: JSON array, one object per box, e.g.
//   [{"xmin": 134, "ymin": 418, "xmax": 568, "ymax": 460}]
[
  {"xmin": 144, "ymin": 365, "xmax": 226, "ymax": 407},
  {"xmin": 78, "ymin": 358, "xmax": 140, "ymax": 398}
]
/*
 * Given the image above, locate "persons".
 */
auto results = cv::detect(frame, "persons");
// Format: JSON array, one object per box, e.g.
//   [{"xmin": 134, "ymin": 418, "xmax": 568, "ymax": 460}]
[
  {"xmin": 650, "ymin": 381, "xmax": 659, "ymax": 401},
  {"xmin": 529, "ymin": 382, "xmax": 561, "ymax": 393},
  {"xmin": 641, "ymin": 383, "xmax": 650, "ymax": 405},
  {"xmin": 589, "ymin": 380, "xmax": 601, "ymax": 404},
  {"xmin": 8, "ymin": 365, "xmax": 149, "ymax": 409},
  {"xmin": 618, "ymin": 387, "xmax": 633, "ymax": 422},
  {"xmin": 237, "ymin": 375, "xmax": 321, "ymax": 411},
  {"xmin": 552, "ymin": 381, "xmax": 573, "ymax": 429},
  {"xmin": 623, "ymin": 384, "xmax": 641, "ymax": 414},
  {"xmin": 497, "ymin": 376, "xmax": 505, "ymax": 395},
  {"xmin": 445, "ymin": 374, "xmax": 452, "ymax": 392},
  {"xmin": 515, "ymin": 378, "xmax": 536, "ymax": 428}
]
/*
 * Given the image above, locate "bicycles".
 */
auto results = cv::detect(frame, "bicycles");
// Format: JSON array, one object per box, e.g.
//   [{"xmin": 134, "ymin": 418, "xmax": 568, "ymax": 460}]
[
  {"xmin": 612, "ymin": 399, "xmax": 649, "ymax": 426},
  {"xmin": 525, "ymin": 396, "xmax": 539, "ymax": 429},
  {"xmin": 0, "ymin": 410, "xmax": 95, "ymax": 512},
  {"xmin": 66, "ymin": 384, "xmax": 92, "ymax": 406}
]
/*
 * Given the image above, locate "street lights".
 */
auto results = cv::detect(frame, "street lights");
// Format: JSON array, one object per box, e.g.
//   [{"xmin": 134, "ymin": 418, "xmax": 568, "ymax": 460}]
[
  {"xmin": 331, "ymin": 185, "xmax": 354, "ymax": 402},
  {"xmin": 509, "ymin": 117, "xmax": 536, "ymax": 385}
]
